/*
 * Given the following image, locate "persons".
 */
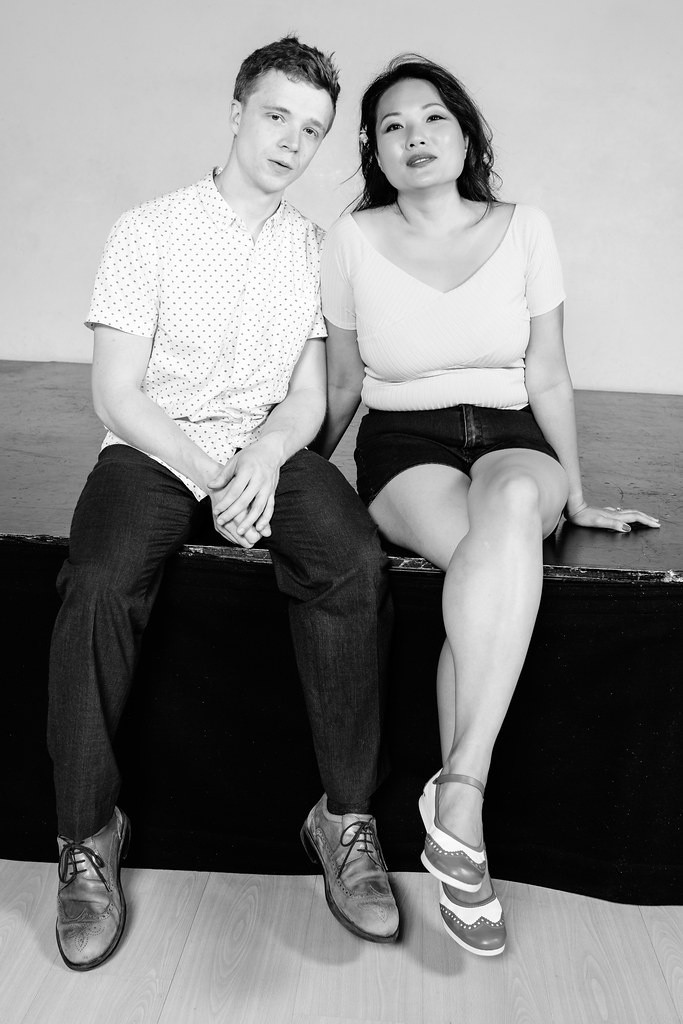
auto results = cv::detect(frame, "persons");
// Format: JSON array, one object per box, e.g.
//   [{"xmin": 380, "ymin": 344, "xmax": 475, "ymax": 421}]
[
  {"xmin": 304, "ymin": 49, "xmax": 662, "ymax": 958},
  {"xmin": 44, "ymin": 33, "xmax": 404, "ymax": 972}
]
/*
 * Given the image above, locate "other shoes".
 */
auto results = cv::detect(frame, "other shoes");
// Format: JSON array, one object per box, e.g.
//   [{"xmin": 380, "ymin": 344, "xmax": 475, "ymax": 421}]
[
  {"xmin": 418, "ymin": 768, "xmax": 488, "ymax": 893},
  {"xmin": 438, "ymin": 879, "xmax": 506, "ymax": 956}
]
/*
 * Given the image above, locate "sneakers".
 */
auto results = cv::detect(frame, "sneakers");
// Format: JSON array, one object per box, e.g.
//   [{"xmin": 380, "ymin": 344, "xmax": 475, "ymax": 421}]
[
  {"xmin": 300, "ymin": 793, "xmax": 400, "ymax": 944},
  {"xmin": 56, "ymin": 806, "xmax": 131, "ymax": 972}
]
